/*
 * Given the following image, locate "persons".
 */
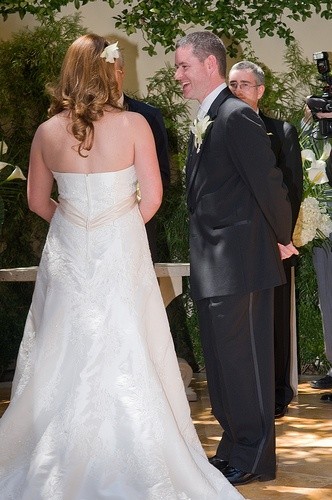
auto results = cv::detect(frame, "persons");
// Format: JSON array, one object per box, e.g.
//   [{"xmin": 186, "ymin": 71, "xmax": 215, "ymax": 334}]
[
  {"xmin": 299, "ymin": 105, "xmax": 332, "ymax": 402},
  {"xmin": 112, "ymin": 54, "xmax": 171, "ymax": 264},
  {"xmin": 226, "ymin": 61, "xmax": 303, "ymax": 418},
  {"xmin": 173, "ymin": 32, "xmax": 300, "ymax": 486},
  {"xmin": 0, "ymin": 31, "xmax": 246, "ymax": 500}
]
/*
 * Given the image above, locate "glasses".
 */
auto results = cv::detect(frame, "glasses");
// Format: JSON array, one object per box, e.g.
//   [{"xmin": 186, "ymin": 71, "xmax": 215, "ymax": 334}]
[{"xmin": 229, "ymin": 83, "xmax": 260, "ymax": 90}]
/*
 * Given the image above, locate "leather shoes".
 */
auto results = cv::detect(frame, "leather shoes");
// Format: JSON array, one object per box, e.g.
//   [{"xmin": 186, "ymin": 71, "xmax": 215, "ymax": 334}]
[
  {"xmin": 274, "ymin": 405, "xmax": 288, "ymax": 418},
  {"xmin": 208, "ymin": 454, "xmax": 229, "ymax": 471},
  {"xmin": 320, "ymin": 393, "xmax": 332, "ymax": 400},
  {"xmin": 311, "ymin": 375, "xmax": 332, "ymax": 389},
  {"xmin": 222, "ymin": 464, "xmax": 276, "ymax": 486}
]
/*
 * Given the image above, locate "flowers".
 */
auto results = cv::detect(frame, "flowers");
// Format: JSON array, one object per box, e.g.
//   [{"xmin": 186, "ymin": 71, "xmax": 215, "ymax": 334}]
[
  {"xmin": 100, "ymin": 41, "xmax": 119, "ymax": 63},
  {"xmin": 189, "ymin": 115, "xmax": 215, "ymax": 154}
]
